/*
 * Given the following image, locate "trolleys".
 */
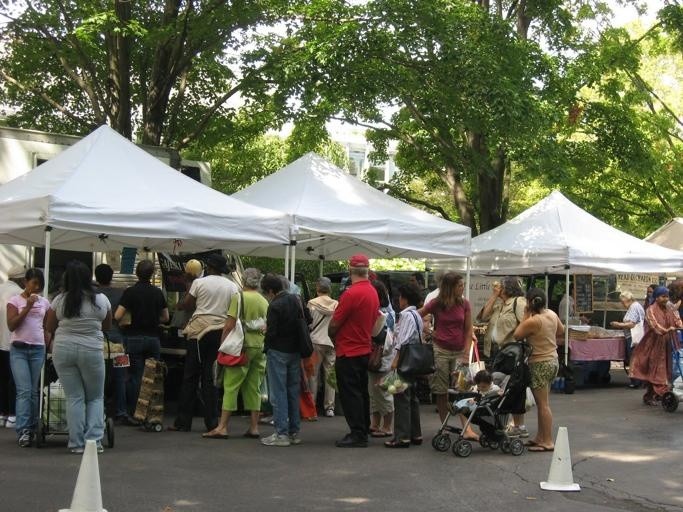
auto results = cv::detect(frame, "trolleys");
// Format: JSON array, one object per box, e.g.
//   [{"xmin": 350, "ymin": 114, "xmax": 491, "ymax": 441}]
[
  {"xmin": 34, "ymin": 327, "xmax": 114, "ymax": 448},
  {"xmin": 133, "ymin": 356, "xmax": 167, "ymax": 432}
]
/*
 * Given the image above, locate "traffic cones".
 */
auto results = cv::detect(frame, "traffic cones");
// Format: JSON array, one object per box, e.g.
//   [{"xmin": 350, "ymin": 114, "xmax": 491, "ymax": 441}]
[
  {"xmin": 539, "ymin": 425, "xmax": 581, "ymax": 491},
  {"xmin": 57, "ymin": 440, "xmax": 108, "ymax": 511}
]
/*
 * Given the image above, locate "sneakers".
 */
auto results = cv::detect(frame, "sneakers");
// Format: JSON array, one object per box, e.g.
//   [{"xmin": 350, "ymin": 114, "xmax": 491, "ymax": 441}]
[
  {"xmin": 506, "ymin": 426, "xmax": 529, "ymax": 438},
  {"xmin": 260, "ymin": 433, "xmax": 290, "ymax": 447},
  {"xmin": 0, "ymin": 416, "xmax": 104, "ymax": 455},
  {"xmin": 259, "ymin": 409, "xmax": 335, "ymax": 426},
  {"xmin": 291, "ymin": 433, "xmax": 301, "ymax": 445}
]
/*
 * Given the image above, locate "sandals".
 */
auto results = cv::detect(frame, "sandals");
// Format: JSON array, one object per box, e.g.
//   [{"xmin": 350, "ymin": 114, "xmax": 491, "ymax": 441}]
[
  {"xmin": 384, "ymin": 439, "xmax": 411, "ymax": 448},
  {"xmin": 411, "ymin": 435, "xmax": 423, "ymax": 444}
]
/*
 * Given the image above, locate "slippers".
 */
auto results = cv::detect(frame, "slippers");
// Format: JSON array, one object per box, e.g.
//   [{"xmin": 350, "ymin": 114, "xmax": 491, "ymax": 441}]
[
  {"xmin": 523, "ymin": 440, "xmax": 538, "ymax": 447},
  {"xmin": 371, "ymin": 429, "xmax": 393, "ymax": 437},
  {"xmin": 201, "ymin": 428, "xmax": 230, "ymax": 439},
  {"xmin": 244, "ymin": 429, "xmax": 260, "ymax": 438},
  {"xmin": 527, "ymin": 442, "xmax": 556, "ymax": 453},
  {"xmin": 462, "ymin": 430, "xmax": 479, "ymax": 441}
]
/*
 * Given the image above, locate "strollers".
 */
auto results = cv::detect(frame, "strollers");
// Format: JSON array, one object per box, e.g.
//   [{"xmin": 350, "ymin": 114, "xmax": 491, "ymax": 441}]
[
  {"xmin": 661, "ymin": 328, "xmax": 683, "ymax": 413},
  {"xmin": 431, "ymin": 340, "xmax": 533, "ymax": 457}
]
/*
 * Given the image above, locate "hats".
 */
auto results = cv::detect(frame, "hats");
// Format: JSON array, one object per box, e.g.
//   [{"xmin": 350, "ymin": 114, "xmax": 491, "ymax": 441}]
[
  {"xmin": 653, "ymin": 285, "xmax": 670, "ymax": 299},
  {"xmin": 349, "ymin": 255, "xmax": 370, "ymax": 268},
  {"xmin": 200, "ymin": 253, "xmax": 231, "ymax": 274}
]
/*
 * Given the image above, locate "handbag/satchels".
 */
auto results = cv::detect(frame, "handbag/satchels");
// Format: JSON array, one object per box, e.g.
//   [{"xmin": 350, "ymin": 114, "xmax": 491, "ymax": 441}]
[
  {"xmin": 366, "ymin": 343, "xmax": 384, "ymax": 373},
  {"xmin": 277, "ymin": 318, "xmax": 313, "ymax": 359},
  {"xmin": 216, "ymin": 290, "xmax": 249, "ymax": 367},
  {"xmin": 470, "ymin": 341, "xmax": 485, "ymax": 377},
  {"xmin": 397, "ymin": 345, "xmax": 436, "ymax": 376},
  {"xmin": 514, "ymin": 341, "xmax": 530, "ymax": 385},
  {"xmin": 218, "ymin": 293, "xmax": 245, "ymax": 358}
]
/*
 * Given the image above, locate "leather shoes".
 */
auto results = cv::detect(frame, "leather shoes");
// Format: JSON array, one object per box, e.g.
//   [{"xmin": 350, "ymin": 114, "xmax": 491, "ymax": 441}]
[
  {"xmin": 335, "ymin": 438, "xmax": 370, "ymax": 448},
  {"xmin": 167, "ymin": 425, "xmax": 191, "ymax": 432}
]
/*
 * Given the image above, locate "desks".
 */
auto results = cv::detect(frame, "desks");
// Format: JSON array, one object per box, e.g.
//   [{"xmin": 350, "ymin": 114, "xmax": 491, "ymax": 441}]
[{"xmin": 556, "ymin": 336, "xmax": 627, "ymax": 387}]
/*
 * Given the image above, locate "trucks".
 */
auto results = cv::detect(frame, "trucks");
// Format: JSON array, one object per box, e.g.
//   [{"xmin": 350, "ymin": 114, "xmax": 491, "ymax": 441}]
[{"xmin": 0, "ymin": 124, "xmax": 244, "ymax": 291}]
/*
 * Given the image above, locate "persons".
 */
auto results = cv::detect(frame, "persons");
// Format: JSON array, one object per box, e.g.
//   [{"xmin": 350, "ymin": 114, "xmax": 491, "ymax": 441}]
[
  {"xmin": 609, "ymin": 280, "xmax": 683, "ymax": 406},
  {"xmin": 1, "ymin": 254, "xmax": 589, "ymax": 454}
]
